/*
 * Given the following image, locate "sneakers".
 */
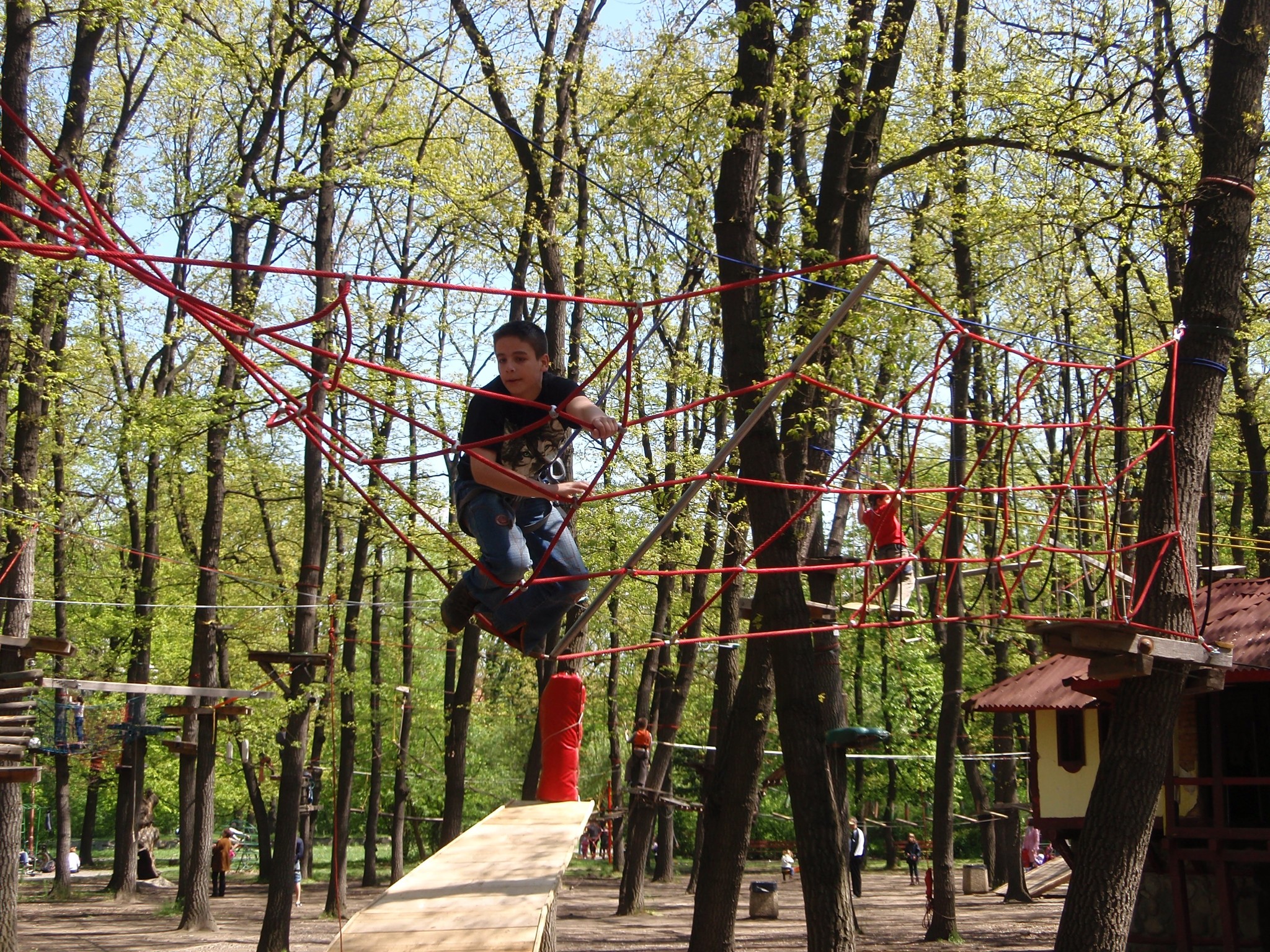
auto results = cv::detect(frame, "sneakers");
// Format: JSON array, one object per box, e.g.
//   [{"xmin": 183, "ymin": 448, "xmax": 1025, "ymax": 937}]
[
  {"xmin": 476, "ymin": 608, "xmax": 542, "ymax": 659},
  {"xmin": 441, "ymin": 572, "xmax": 480, "ymax": 634}
]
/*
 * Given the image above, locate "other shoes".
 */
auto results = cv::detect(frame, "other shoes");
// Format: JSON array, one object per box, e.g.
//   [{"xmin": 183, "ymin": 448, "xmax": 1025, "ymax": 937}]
[
  {"xmin": 890, "ymin": 605, "xmax": 916, "ymax": 618},
  {"xmin": 210, "ymin": 895, "xmax": 224, "ymax": 897},
  {"xmin": 295, "ymin": 902, "xmax": 301, "ymax": 907},
  {"xmin": 789, "ymin": 875, "xmax": 793, "ymax": 880}
]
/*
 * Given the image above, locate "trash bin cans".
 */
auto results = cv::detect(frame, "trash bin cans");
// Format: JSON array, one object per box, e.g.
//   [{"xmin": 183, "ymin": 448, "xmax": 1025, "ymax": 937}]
[
  {"xmin": 748, "ymin": 879, "xmax": 780, "ymax": 918},
  {"xmin": 962, "ymin": 863, "xmax": 989, "ymax": 895}
]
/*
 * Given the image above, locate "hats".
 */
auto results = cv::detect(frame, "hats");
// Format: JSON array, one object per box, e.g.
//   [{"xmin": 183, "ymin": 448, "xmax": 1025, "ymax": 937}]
[{"xmin": 848, "ymin": 818, "xmax": 856, "ymax": 826}]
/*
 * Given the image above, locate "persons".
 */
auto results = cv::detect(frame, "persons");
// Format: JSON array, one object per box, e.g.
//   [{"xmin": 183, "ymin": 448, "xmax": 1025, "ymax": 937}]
[
  {"xmin": 441, "ymin": 322, "xmax": 615, "ymax": 656},
  {"xmin": 210, "ymin": 829, "xmax": 232, "ymax": 897},
  {"xmin": 602, "ymin": 831, "xmax": 613, "ymax": 858},
  {"xmin": 781, "ymin": 849, "xmax": 794, "ymax": 881},
  {"xmin": 304, "ymin": 771, "xmax": 315, "ymax": 805},
  {"xmin": 624, "ymin": 717, "xmax": 653, "ymax": 788},
  {"xmin": 69, "ymin": 695, "xmax": 85, "ymax": 744},
  {"xmin": 228, "ymin": 822, "xmax": 250, "ymax": 851},
  {"xmin": 294, "ymin": 837, "xmax": 304, "ymax": 907},
  {"xmin": 586, "ymin": 821, "xmax": 600, "ymax": 859},
  {"xmin": 857, "ymin": 481, "xmax": 916, "ymax": 621},
  {"xmin": 905, "ymin": 833, "xmax": 921, "ymax": 885},
  {"xmin": 581, "ymin": 840, "xmax": 589, "ymax": 859},
  {"xmin": 1023, "ymin": 819, "xmax": 1039, "ymax": 871},
  {"xmin": 68, "ymin": 847, "xmax": 80, "ymax": 873},
  {"xmin": 20, "ymin": 851, "xmax": 33, "ymax": 868},
  {"xmin": 256, "ymin": 752, "xmax": 275, "ymax": 787},
  {"xmin": 849, "ymin": 817, "xmax": 864, "ymax": 899}
]
